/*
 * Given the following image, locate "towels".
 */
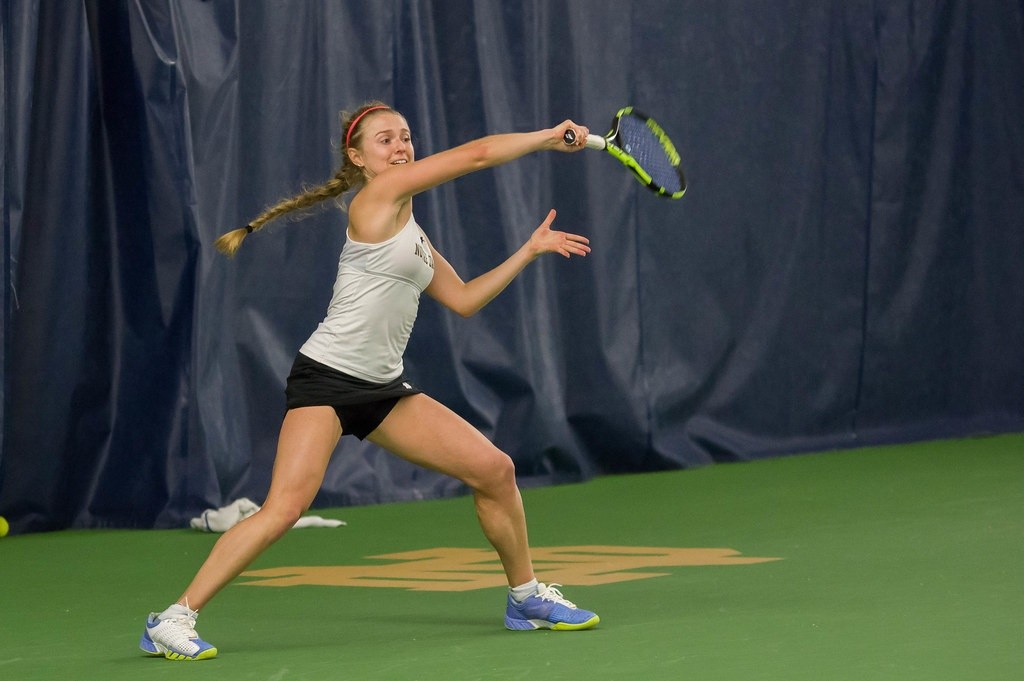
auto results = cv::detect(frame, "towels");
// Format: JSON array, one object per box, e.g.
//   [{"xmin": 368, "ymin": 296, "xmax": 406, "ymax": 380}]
[{"xmin": 190, "ymin": 497, "xmax": 347, "ymax": 533}]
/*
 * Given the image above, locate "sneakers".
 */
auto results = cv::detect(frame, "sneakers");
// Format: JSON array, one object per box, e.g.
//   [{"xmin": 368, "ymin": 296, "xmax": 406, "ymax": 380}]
[
  {"xmin": 139, "ymin": 597, "xmax": 217, "ymax": 661},
  {"xmin": 504, "ymin": 582, "xmax": 600, "ymax": 631}
]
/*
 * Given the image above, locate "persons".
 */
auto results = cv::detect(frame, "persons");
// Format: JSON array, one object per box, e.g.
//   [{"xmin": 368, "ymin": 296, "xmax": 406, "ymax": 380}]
[{"xmin": 137, "ymin": 98, "xmax": 603, "ymax": 660}]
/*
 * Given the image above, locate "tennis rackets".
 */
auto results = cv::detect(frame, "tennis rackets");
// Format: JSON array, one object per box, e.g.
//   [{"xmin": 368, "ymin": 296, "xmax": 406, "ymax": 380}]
[{"xmin": 564, "ymin": 97, "xmax": 692, "ymax": 208}]
